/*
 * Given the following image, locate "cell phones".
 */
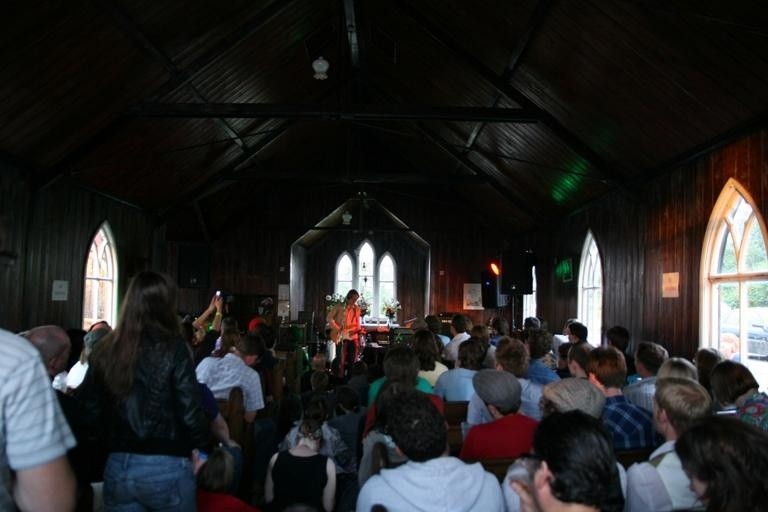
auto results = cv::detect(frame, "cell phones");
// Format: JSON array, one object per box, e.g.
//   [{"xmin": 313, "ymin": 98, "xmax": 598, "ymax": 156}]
[{"xmin": 215, "ymin": 291, "xmax": 221, "ymax": 299}]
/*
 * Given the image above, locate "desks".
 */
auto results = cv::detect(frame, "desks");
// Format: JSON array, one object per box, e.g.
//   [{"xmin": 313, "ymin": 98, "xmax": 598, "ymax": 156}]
[{"xmin": 357, "ymin": 324, "xmax": 400, "ymax": 340}]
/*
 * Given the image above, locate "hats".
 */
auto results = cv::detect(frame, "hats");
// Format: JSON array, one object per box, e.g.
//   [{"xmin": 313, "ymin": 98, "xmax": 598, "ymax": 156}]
[
  {"xmin": 541, "ymin": 378, "xmax": 605, "ymax": 418},
  {"xmin": 473, "ymin": 369, "xmax": 522, "ymax": 407}
]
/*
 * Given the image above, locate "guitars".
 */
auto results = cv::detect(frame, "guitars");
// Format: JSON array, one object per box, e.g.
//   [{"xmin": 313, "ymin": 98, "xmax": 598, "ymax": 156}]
[{"xmin": 331, "ymin": 325, "xmax": 390, "ymax": 344}]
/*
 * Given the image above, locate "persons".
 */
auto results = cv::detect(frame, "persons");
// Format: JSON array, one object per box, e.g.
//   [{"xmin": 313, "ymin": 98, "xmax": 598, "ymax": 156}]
[{"xmin": 0, "ymin": 269, "xmax": 767, "ymax": 512}]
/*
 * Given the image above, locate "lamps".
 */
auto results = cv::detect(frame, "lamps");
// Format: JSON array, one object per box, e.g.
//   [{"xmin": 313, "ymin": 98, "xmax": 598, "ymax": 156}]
[
  {"xmin": 308, "ymin": 0, "xmax": 330, "ymax": 85},
  {"xmin": 339, "ymin": 202, "xmax": 356, "ymax": 229}
]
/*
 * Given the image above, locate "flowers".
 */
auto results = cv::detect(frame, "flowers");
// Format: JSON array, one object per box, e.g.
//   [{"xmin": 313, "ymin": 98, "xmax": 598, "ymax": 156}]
[{"xmin": 324, "ymin": 292, "xmax": 403, "ymax": 324}]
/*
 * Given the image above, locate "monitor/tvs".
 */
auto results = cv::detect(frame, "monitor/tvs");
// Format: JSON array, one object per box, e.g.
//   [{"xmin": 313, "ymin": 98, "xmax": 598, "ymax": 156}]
[{"xmin": 179, "ymin": 246, "xmax": 210, "ymax": 288}]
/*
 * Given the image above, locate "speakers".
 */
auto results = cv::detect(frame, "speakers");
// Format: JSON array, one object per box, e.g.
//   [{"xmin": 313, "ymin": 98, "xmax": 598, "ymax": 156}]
[
  {"xmin": 482, "ymin": 272, "xmax": 509, "ymax": 308},
  {"xmin": 503, "ymin": 255, "xmax": 532, "ymax": 293}
]
